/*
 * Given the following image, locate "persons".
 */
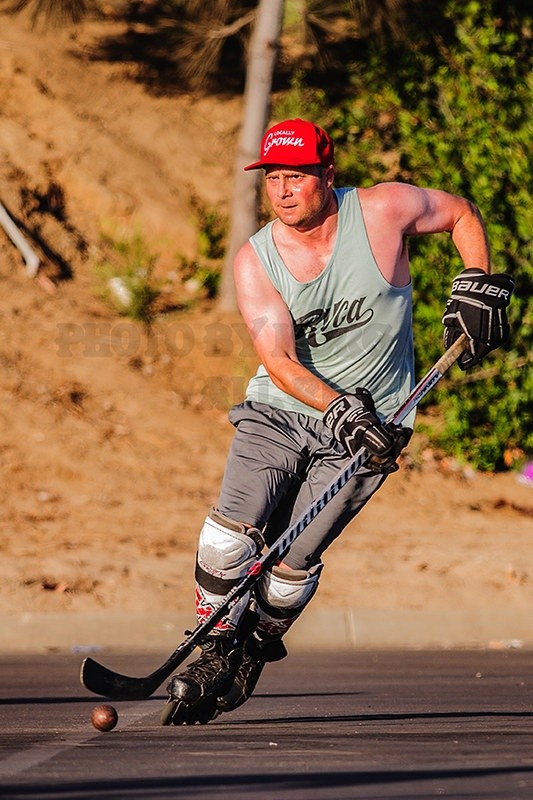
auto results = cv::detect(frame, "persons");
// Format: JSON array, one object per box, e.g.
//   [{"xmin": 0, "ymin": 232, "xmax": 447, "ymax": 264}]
[{"xmin": 160, "ymin": 118, "xmax": 514, "ymax": 725}]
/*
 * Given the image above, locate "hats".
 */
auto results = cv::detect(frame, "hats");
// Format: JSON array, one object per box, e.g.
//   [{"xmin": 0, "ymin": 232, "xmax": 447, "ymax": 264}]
[{"xmin": 243, "ymin": 118, "xmax": 335, "ymax": 171}]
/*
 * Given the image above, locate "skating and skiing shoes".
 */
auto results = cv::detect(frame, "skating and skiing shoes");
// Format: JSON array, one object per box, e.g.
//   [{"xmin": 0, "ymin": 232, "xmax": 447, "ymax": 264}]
[
  {"xmin": 213, "ymin": 580, "xmax": 319, "ymax": 720},
  {"xmin": 159, "ymin": 609, "xmax": 261, "ymax": 724}
]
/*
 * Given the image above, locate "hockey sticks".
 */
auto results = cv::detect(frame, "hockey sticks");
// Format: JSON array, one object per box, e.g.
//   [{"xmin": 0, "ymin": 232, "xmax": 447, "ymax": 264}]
[{"xmin": 80, "ymin": 333, "xmax": 469, "ymax": 700}]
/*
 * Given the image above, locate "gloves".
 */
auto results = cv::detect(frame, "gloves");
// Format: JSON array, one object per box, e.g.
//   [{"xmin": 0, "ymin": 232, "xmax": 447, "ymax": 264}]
[
  {"xmin": 323, "ymin": 387, "xmax": 409, "ymax": 474},
  {"xmin": 442, "ymin": 270, "xmax": 515, "ymax": 371}
]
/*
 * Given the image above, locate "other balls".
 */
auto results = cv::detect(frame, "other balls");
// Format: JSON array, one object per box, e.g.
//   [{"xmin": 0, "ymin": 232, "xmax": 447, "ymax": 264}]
[{"xmin": 91, "ymin": 705, "xmax": 118, "ymax": 732}]
